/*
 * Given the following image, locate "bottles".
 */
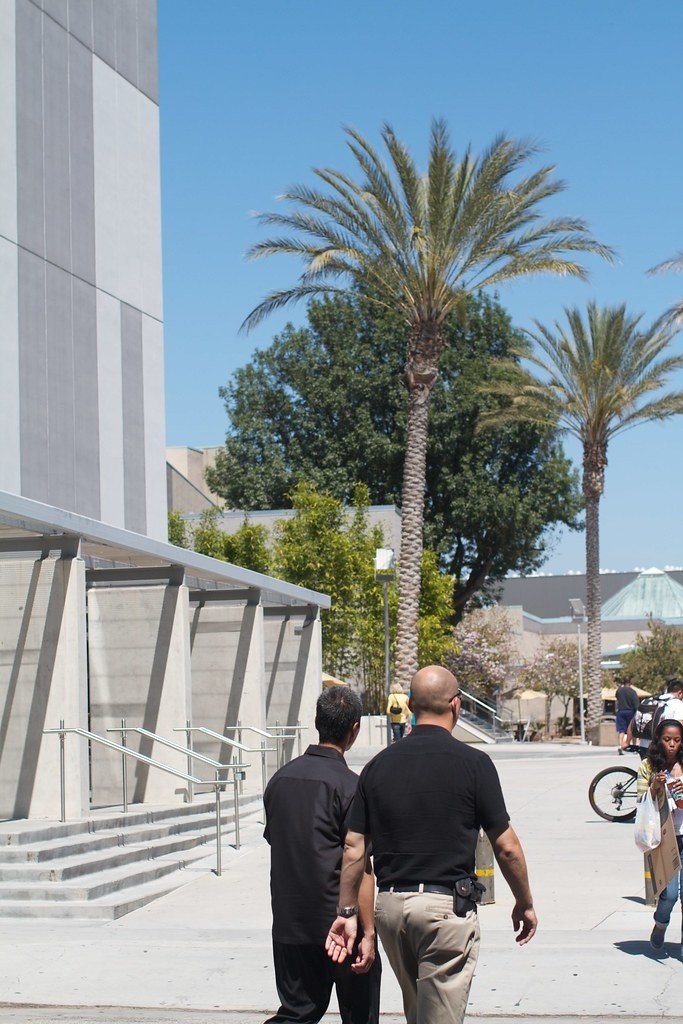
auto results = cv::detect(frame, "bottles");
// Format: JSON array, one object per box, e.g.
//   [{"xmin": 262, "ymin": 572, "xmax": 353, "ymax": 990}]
[{"xmin": 664, "ymin": 770, "xmax": 683, "ymax": 809}]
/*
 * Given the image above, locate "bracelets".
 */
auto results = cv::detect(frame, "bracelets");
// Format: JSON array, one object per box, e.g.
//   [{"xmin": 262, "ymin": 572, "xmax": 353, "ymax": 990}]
[
  {"xmin": 650, "ymin": 782, "xmax": 660, "ymax": 791},
  {"xmin": 336, "ymin": 905, "xmax": 359, "ymax": 916}
]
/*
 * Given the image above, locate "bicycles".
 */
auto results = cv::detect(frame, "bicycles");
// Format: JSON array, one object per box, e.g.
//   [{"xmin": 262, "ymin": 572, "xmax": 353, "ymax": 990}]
[{"xmin": 587, "ymin": 744, "xmax": 652, "ymax": 822}]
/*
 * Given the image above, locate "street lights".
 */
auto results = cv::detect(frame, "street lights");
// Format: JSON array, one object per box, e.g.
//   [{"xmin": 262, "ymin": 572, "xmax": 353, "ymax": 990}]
[
  {"xmin": 371, "ymin": 548, "xmax": 398, "ymax": 747},
  {"xmin": 568, "ymin": 598, "xmax": 588, "ymax": 741}
]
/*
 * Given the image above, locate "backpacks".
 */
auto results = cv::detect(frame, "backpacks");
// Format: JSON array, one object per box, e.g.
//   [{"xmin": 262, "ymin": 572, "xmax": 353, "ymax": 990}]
[{"xmin": 632, "ymin": 694, "xmax": 677, "ymax": 739}]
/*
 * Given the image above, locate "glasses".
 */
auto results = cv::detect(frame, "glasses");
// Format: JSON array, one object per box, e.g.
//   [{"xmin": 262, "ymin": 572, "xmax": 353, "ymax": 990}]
[{"xmin": 448, "ymin": 690, "xmax": 462, "ymax": 703}]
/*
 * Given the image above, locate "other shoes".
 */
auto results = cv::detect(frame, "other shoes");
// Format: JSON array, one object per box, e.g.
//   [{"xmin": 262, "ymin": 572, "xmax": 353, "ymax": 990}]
[
  {"xmin": 618, "ymin": 747, "xmax": 624, "ymax": 755},
  {"xmin": 651, "ymin": 925, "xmax": 666, "ymax": 951}
]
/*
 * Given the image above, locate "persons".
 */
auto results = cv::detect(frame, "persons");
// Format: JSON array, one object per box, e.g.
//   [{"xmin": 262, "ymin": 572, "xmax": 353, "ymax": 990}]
[
  {"xmin": 615, "ymin": 675, "xmax": 640, "ymax": 755},
  {"xmin": 386, "ymin": 683, "xmax": 416, "ymax": 742},
  {"xmin": 263, "ymin": 685, "xmax": 382, "ymax": 1024},
  {"xmin": 636, "ymin": 719, "xmax": 683, "ymax": 951},
  {"xmin": 621, "ymin": 675, "xmax": 683, "ymax": 761},
  {"xmin": 323, "ymin": 664, "xmax": 537, "ymax": 1024}
]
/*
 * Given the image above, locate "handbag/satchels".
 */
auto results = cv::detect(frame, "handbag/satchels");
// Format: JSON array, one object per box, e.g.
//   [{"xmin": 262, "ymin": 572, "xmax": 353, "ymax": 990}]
[
  {"xmin": 635, "ymin": 787, "xmax": 662, "ymax": 853},
  {"xmin": 391, "ymin": 693, "xmax": 402, "ymax": 714}
]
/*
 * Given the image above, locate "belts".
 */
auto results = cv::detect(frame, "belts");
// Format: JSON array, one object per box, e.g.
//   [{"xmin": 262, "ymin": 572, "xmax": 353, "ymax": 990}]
[{"xmin": 379, "ymin": 884, "xmax": 454, "ymax": 895}]
